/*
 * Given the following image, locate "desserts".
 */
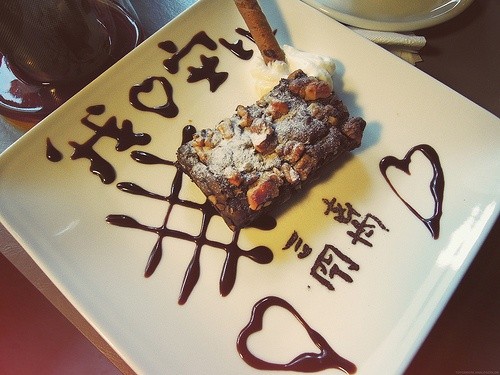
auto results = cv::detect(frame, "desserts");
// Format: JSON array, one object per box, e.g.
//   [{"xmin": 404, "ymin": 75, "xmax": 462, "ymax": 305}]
[{"xmin": 176, "ymin": 45, "xmax": 367, "ymax": 233}]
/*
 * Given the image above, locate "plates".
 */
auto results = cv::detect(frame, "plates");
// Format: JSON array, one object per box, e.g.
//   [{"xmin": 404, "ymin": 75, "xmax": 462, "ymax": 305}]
[{"xmin": 0, "ymin": 1, "xmax": 500, "ymax": 375}]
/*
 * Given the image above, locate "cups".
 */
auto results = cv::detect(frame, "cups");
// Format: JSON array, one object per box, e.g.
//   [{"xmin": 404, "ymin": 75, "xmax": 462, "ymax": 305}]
[{"xmin": 1, "ymin": 0, "xmax": 113, "ymax": 87}]
